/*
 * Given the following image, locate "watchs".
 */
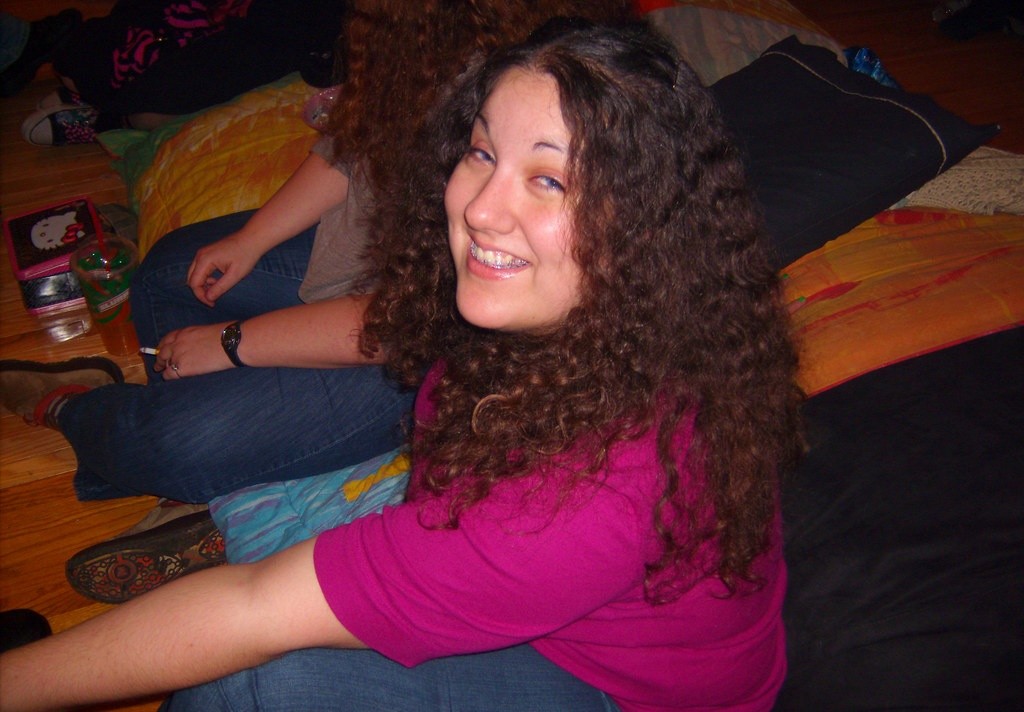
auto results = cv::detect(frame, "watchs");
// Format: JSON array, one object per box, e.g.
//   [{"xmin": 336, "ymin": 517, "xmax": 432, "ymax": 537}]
[{"xmin": 221, "ymin": 321, "xmax": 247, "ymax": 367}]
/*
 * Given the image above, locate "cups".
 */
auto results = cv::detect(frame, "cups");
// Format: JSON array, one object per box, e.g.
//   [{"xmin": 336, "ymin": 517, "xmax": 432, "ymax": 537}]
[{"xmin": 69, "ymin": 231, "xmax": 141, "ymax": 358}]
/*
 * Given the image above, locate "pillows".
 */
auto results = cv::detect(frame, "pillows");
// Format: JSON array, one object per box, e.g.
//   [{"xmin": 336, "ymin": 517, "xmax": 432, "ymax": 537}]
[{"xmin": 706, "ymin": 34, "xmax": 1000, "ymax": 270}]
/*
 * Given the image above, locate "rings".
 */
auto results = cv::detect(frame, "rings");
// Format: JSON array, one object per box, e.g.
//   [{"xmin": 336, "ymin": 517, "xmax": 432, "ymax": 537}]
[{"xmin": 171, "ymin": 365, "xmax": 182, "ymax": 378}]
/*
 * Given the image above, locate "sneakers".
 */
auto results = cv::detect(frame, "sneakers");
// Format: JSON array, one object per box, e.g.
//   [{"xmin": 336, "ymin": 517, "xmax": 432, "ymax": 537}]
[{"xmin": 20, "ymin": 85, "xmax": 105, "ymax": 145}]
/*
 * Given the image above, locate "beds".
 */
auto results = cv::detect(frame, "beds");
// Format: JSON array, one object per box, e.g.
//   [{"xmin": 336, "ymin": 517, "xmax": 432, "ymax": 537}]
[{"xmin": 97, "ymin": 7, "xmax": 1024, "ymax": 712}]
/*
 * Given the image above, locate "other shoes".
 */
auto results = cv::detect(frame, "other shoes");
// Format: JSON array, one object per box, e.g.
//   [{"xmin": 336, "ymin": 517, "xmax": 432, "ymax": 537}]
[
  {"xmin": 1, "ymin": 356, "xmax": 125, "ymax": 428},
  {"xmin": 0, "ymin": 608, "xmax": 53, "ymax": 654},
  {"xmin": 65, "ymin": 509, "xmax": 226, "ymax": 604}
]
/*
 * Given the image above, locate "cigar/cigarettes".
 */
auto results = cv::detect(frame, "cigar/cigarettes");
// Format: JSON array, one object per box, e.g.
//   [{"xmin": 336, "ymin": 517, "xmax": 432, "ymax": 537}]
[{"xmin": 139, "ymin": 347, "xmax": 160, "ymax": 354}]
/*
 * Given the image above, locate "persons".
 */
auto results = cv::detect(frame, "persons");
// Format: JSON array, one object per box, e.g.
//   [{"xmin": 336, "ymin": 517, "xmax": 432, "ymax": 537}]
[
  {"xmin": 21, "ymin": 0, "xmax": 352, "ymax": 146},
  {"xmin": 0, "ymin": 0, "xmax": 659, "ymax": 502},
  {"xmin": 0, "ymin": 15, "xmax": 797, "ymax": 712}
]
